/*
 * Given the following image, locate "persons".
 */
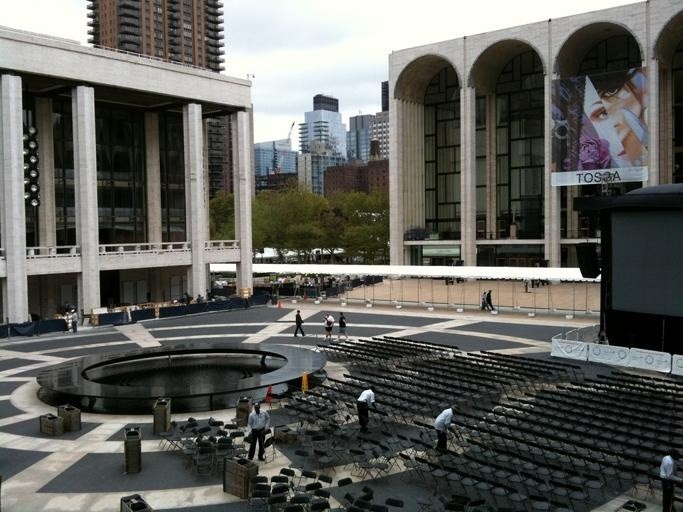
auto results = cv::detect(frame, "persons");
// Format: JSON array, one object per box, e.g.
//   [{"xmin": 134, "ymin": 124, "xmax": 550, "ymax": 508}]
[
  {"xmin": 67, "ymin": 310, "xmax": 77, "ymax": 332},
  {"xmin": 355, "ymin": 385, "xmax": 379, "ymax": 434},
  {"xmin": 432, "ymin": 403, "xmax": 462, "ymax": 453},
  {"xmin": 245, "ymin": 401, "xmax": 269, "ymax": 461},
  {"xmin": 196, "ymin": 294, "xmax": 202, "ymax": 303},
  {"xmin": 323, "ymin": 313, "xmax": 332, "ymax": 341},
  {"xmin": 332, "ymin": 312, "xmax": 349, "ymax": 342},
  {"xmin": 486, "ymin": 289, "xmax": 495, "ymax": 310},
  {"xmin": 480, "ymin": 291, "xmax": 490, "ymax": 311},
  {"xmin": 292, "ymin": 309, "xmax": 305, "ymax": 337},
  {"xmin": 29, "ymin": 311, "xmax": 41, "ymax": 334}
]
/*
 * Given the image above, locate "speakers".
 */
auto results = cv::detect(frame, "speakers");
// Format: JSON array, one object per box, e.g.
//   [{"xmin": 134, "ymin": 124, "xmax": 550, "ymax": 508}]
[{"xmin": 576, "ymin": 243, "xmax": 600, "ymax": 278}]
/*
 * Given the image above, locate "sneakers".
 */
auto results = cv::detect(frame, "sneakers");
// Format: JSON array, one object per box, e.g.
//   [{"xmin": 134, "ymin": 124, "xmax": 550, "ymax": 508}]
[
  {"xmin": 258, "ymin": 456, "xmax": 266, "ymax": 461},
  {"xmin": 358, "ymin": 428, "xmax": 452, "ymax": 456},
  {"xmin": 248, "ymin": 458, "xmax": 253, "ymax": 460},
  {"xmin": 481, "ymin": 307, "xmax": 495, "ymax": 310},
  {"xmin": 293, "ymin": 333, "xmax": 349, "ymax": 341}
]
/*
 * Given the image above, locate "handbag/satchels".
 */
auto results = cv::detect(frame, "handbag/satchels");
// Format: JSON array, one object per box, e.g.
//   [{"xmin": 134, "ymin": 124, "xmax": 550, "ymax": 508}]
[{"xmin": 325, "ymin": 318, "xmax": 332, "ymax": 326}]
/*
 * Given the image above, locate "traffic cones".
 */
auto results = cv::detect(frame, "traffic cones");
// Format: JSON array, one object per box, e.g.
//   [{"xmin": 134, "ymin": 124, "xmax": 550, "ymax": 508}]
[
  {"xmin": 276, "ymin": 300, "xmax": 281, "ymax": 309},
  {"xmin": 302, "ymin": 290, "xmax": 307, "ymax": 299},
  {"xmin": 264, "ymin": 385, "xmax": 275, "ymax": 402},
  {"xmin": 300, "ymin": 371, "xmax": 308, "ymax": 392}
]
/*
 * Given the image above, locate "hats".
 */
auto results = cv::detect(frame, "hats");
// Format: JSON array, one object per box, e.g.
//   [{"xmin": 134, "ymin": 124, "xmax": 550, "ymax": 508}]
[
  {"xmin": 451, "ymin": 404, "xmax": 466, "ymax": 415},
  {"xmin": 254, "ymin": 401, "xmax": 260, "ymax": 407}
]
